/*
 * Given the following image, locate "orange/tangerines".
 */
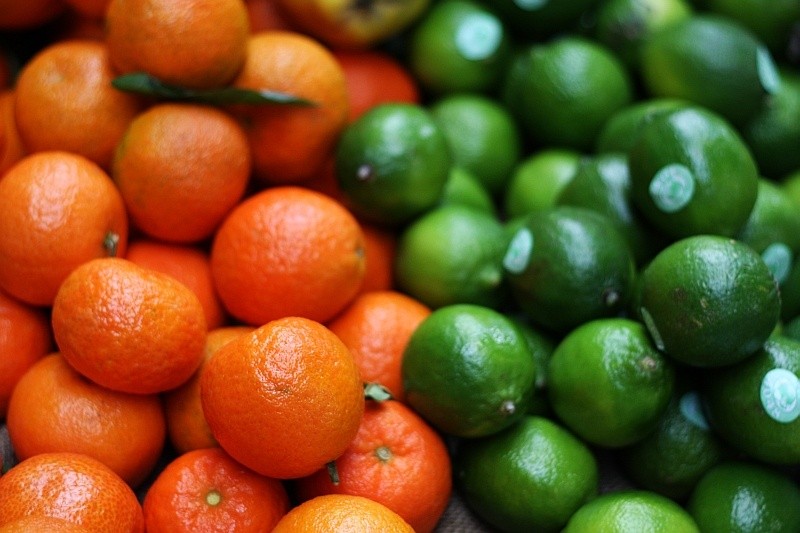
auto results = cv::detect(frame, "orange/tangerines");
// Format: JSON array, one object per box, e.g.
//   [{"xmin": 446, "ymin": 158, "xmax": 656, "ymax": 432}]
[{"xmin": 0, "ymin": 0, "xmax": 452, "ymax": 533}]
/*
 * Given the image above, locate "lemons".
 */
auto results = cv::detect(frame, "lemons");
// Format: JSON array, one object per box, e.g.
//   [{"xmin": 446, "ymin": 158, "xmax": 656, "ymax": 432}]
[{"xmin": 331, "ymin": 1, "xmax": 800, "ymax": 533}]
